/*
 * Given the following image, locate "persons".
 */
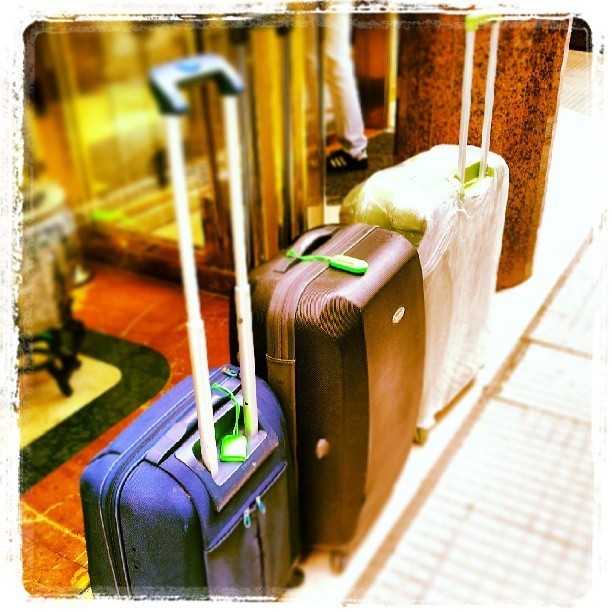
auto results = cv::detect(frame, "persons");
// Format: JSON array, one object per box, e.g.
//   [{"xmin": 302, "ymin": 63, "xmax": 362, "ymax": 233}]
[{"xmin": 321, "ymin": 13, "xmax": 368, "ymax": 175}]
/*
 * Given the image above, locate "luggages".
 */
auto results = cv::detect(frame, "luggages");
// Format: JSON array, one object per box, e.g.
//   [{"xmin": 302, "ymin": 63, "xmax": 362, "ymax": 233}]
[
  {"xmin": 339, "ymin": 5, "xmax": 509, "ymax": 446},
  {"xmin": 80, "ymin": 54, "xmax": 304, "ymax": 602},
  {"xmin": 228, "ymin": 222, "xmax": 426, "ymax": 576}
]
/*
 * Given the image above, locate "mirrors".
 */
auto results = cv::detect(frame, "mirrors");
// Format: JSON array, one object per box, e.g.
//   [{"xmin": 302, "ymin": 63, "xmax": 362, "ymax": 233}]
[
  {"xmin": 268, "ymin": 20, "xmax": 400, "ymax": 260},
  {"xmin": 35, "ymin": 24, "xmax": 233, "ymax": 294}
]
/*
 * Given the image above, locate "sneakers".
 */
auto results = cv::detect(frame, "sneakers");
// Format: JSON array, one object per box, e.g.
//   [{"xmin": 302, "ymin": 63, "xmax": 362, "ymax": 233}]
[{"xmin": 326, "ymin": 148, "xmax": 369, "ymax": 174}]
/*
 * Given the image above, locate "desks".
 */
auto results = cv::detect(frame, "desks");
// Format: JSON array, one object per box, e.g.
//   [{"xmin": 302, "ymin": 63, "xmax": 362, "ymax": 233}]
[{"xmin": 21, "ymin": 183, "xmax": 82, "ymax": 396}]
